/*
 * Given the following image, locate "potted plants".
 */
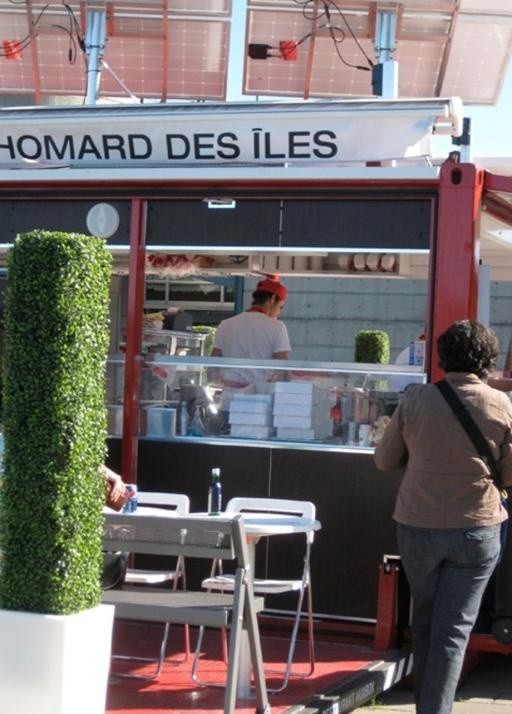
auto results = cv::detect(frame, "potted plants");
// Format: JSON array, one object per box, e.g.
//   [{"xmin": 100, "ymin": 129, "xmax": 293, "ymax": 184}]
[{"xmin": 1, "ymin": 228, "xmax": 116, "ymax": 712}]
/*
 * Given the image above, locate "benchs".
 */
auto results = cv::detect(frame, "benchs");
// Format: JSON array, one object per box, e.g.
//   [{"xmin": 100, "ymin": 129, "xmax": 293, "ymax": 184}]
[{"xmin": 101, "ymin": 512, "xmax": 271, "ymax": 714}]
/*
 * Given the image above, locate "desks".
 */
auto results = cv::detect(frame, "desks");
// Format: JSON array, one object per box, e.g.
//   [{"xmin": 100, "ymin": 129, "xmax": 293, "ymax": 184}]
[{"xmin": 103, "ymin": 512, "xmax": 322, "ymax": 700}]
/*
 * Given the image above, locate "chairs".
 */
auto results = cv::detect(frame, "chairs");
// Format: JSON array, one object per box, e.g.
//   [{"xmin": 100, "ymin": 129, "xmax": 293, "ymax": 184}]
[
  {"xmin": 103, "ymin": 492, "xmax": 190, "ymax": 681},
  {"xmin": 192, "ymin": 497, "xmax": 316, "ymax": 694}
]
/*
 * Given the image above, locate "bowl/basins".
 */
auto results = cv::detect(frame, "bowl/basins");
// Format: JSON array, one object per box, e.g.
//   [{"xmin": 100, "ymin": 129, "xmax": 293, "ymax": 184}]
[{"xmin": 146, "ymin": 345, "xmax": 190, "ymax": 358}]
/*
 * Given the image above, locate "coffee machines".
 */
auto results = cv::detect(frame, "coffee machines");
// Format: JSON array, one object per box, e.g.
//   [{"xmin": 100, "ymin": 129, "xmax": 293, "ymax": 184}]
[{"xmin": 335, "ymin": 386, "xmax": 399, "ymax": 447}]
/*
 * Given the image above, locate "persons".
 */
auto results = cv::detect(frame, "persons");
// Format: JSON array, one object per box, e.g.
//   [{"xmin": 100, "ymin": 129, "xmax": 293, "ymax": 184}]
[
  {"xmin": 373, "ymin": 315, "xmax": 512, "ymax": 714},
  {"xmin": 205, "ymin": 278, "xmax": 293, "ymax": 429},
  {"xmin": 103, "ymin": 466, "xmax": 127, "ymax": 502}
]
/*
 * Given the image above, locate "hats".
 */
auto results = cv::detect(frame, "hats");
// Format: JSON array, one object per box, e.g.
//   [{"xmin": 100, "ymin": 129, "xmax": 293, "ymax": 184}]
[{"xmin": 257, "ymin": 279, "xmax": 286, "ymax": 299}]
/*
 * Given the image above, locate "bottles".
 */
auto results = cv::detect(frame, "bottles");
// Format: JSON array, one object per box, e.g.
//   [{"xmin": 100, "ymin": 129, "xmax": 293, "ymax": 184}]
[
  {"xmin": 179, "ymin": 401, "xmax": 206, "ymax": 436},
  {"xmin": 207, "ymin": 467, "xmax": 222, "ymax": 516},
  {"xmin": 348, "ymin": 422, "xmax": 357, "ymax": 445},
  {"xmin": 358, "ymin": 424, "xmax": 372, "ymax": 449}
]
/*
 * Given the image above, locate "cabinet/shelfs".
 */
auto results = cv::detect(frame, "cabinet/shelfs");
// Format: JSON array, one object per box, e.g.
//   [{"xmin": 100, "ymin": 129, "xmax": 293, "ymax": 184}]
[{"xmin": 105, "ymin": 351, "xmax": 428, "ymax": 461}]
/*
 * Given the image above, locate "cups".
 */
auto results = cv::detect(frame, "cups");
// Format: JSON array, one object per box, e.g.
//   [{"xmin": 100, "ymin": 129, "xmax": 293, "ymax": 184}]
[
  {"xmin": 337, "ymin": 251, "xmax": 396, "ymax": 272},
  {"xmin": 250, "ymin": 253, "xmax": 323, "ymax": 272}
]
